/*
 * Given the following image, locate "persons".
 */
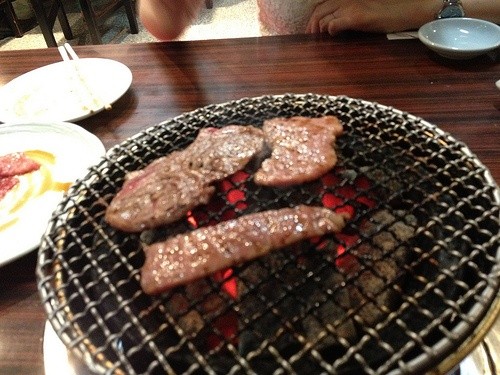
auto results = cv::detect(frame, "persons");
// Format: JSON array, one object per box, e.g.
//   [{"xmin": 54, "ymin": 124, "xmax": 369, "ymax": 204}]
[{"xmin": 137, "ymin": 0, "xmax": 500, "ymax": 42}]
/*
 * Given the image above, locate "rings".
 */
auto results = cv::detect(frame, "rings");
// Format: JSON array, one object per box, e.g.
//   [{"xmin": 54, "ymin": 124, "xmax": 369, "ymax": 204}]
[{"xmin": 331, "ymin": 12, "xmax": 338, "ymax": 19}]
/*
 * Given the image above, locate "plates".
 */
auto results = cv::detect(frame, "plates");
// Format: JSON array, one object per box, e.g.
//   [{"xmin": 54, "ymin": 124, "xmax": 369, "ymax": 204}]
[
  {"xmin": 418, "ymin": 18, "xmax": 500, "ymax": 62},
  {"xmin": 0, "ymin": 119, "xmax": 108, "ymax": 266},
  {"xmin": 0, "ymin": 58, "xmax": 133, "ymax": 123}
]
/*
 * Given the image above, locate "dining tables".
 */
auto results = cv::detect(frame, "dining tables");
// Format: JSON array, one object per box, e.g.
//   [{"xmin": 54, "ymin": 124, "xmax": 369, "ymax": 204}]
[{"xmin": 0, "ymin": 30, "xmax": 500, "ymax": 375}]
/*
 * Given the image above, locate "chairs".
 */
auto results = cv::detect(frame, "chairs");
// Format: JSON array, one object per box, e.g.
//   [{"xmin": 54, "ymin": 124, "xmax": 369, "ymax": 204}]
[{"xmin": 29, "ymin": 0, "xmax": 139, "ymax": 51}]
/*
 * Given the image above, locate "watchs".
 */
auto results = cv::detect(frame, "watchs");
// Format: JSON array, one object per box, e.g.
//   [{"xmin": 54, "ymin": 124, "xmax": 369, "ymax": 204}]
[{"xmin": 435, "ymin": 0, "xmax": 465, "ymax": 20}]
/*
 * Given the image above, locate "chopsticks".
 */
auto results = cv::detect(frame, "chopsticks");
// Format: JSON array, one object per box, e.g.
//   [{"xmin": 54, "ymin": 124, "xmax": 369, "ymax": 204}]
[{"xmin": 58, "ymin": 42, "xmax": 113, "ymax": 115}]
